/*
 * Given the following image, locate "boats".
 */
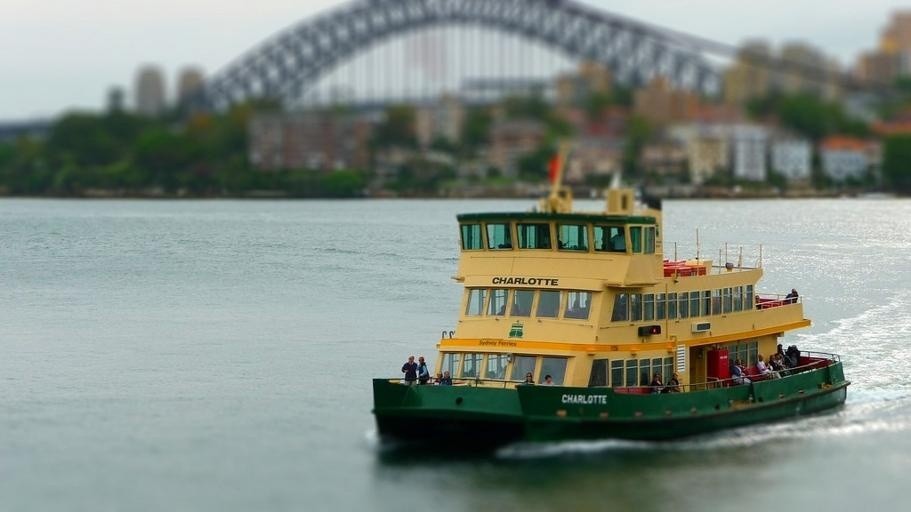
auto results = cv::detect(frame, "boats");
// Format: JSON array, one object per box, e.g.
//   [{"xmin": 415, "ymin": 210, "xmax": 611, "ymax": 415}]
[{"xmin": 372, "ymin": 140, "xmax": 852, "ymax": 458}]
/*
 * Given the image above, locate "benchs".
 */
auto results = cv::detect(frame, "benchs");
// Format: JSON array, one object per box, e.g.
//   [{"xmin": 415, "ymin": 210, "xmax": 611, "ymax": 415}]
[
  {"xmin": 613, "ymin": 386, "xmax": 651, "ymax": 394},
  {"xmin": 745, "ymin": 367, "xmax": 771, "ymax": 381}
]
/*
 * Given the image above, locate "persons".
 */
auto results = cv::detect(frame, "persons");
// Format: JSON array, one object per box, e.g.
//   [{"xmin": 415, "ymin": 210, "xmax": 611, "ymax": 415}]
[
  {"xmin": 786, "ymin": 288, "xmax": 799, "ymax": 304},
  {"xmin": 440, "ymin": 371, "xmax": 452, "ymax": 385},
  {"xmin": 776, "ymin": 352, "xmax": 792, "ymax": 375},
  {"xmin": 668, "ymin": 373, "xmax": 681, "ymax": 392},
  {"xmin": 755, "ymin": 294, "xmax": 764, "ymax": 309},
  {"xmin": 757, "ymin": 354, "xmax": 782, "ymax": 378},
  {"xmin": 741, "ymin": 360, "xmax": 757, "ymax": 381},
  {"xmin": 732, "ymin": 359, "xmax": 752, "ymax": 384},
  {"xmin": 777, "ymin": 343, "xmax": 785, "ymax": 354},
  {"xmin": 610, "ymin": 227, "xmax": 626, "ymax": 250},
  {"xmin": 786, "ymin": 345, "xmax": 803, "ymax": 374},
  {"xmin": 417, "ymin": 356, "xmax": 430, "ymax": 384},
  {"xmin": 769, "ymin": 355, "xmax": 785, "ymax": 377},
  {"xmin": 402, "ymin": 355, "xmax": 419, "ymax": 385},
  {"xmin": 523, "ymin": 372, "xmax": 536, "ymax": 385},
  {"xmin": 542, "ymin": 374, "xmax": 555, "ymax": 385},
  {"xmin": 434, "ymin": 371, "xmax": 444, "ymax": 386},
  {"xmin": 650, "ymin": 371, "xmax": 671, "ymax": 394}
]
